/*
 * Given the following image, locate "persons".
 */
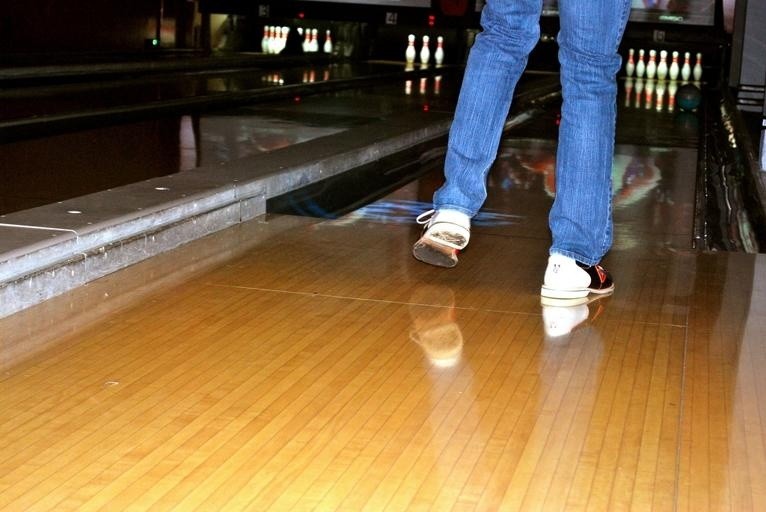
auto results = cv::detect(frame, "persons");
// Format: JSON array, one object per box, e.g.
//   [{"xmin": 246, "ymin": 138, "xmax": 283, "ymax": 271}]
[
  {"xmin": 412, "ymin": 275, "xmax": 606, "ymax": 510},
  {"xmin": 412, "ymin": 0, "xmax": 634, "ymax": 301}
]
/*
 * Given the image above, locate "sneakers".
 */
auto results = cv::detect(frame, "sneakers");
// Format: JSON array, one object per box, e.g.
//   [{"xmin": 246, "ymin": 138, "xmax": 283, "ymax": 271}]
[
  {"xmin": 411, "ymin": 208, "xmax": 471, "ymax": 268},
  {"xmin": 406, "ymin": 282, "xmax": 464, "ymax": 368},
  {"xmin": 540, "ymin": 292, "xmax": 614, "ymax": 338},
  {"xmin": 539, "ymin": 251, "xmax": 614, "ymax": 300}
]
